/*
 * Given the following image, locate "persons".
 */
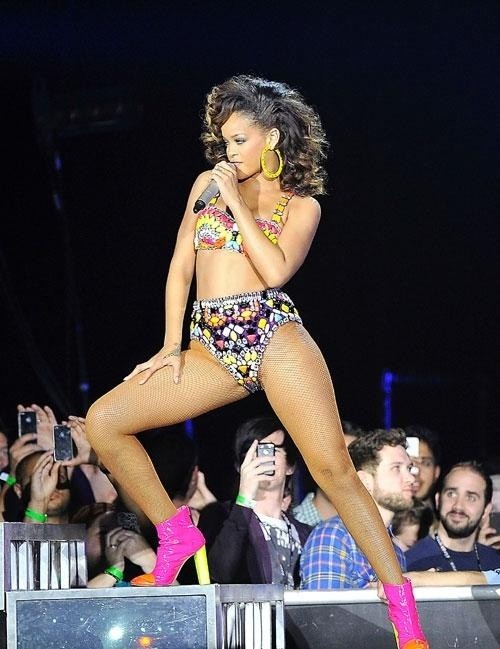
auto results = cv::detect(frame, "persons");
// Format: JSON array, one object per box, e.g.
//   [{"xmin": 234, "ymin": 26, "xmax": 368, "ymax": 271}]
[
  {"xmin": 84, "ymin": 74, "xmax": 431, "ymax": 648},
  {"xmin": 0, "ymin": 405, "xmax": 499, "ymax": 590}
]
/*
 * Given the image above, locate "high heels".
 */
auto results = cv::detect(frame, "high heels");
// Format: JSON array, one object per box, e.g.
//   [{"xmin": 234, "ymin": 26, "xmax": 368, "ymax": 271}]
[
  {"xmin": 130, "ymin": 506, "xmax": 211, "ymax": 587},
  {"xmin": 383, "ymin": 576, "xmax": 429, "ymax": 649}
]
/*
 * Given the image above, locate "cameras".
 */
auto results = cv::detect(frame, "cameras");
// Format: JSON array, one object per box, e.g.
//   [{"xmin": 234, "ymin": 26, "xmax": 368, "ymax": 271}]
[
  {"xmin": 52, "ymin": 424, "xmax": 73, "ymax": 463},
  {"xmin": 405, "ymin": 437, "xmax": 420, "ymax": 476},
  {"xmin": 19, "ymin": 410, "xmax": 37, "ymax": 446},
  {"xmin": 257, "ymin": 443, "xmax": 274, "ymax": 475}
]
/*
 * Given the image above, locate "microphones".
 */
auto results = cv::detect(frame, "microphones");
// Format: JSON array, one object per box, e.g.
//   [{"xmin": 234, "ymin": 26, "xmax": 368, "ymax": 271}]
[{"xmin": 192, "ymin": 162, "xmax": 235, "ymax": 214}]
